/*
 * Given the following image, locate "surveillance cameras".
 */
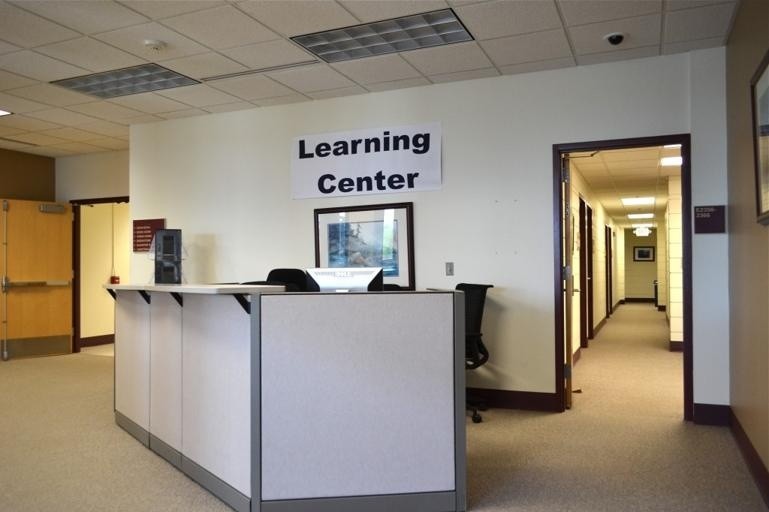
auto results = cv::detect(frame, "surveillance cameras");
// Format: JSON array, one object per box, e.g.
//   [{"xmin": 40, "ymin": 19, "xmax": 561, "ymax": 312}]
[{"xmin": 608, "ymin": 34, "xmax": 624, "ymax": 46}]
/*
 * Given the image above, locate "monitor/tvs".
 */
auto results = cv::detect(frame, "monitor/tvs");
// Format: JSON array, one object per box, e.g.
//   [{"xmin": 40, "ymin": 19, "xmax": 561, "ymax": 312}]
[{"xmin": 305, "ymin": 267, "xmax": 384, "ymax": 292}]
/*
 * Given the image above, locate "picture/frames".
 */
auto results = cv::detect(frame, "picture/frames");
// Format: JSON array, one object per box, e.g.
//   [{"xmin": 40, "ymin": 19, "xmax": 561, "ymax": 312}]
[
  {"xmin": 750, "ymin": 51, "xmax": 769, "ymax": 222},
  {"xmin": 633, "ymin": 246, "xmax": 655, "ymax": 262},
  {"xmin": 314, "ymin": 201, "xmax": 415, "ymax": 290}
]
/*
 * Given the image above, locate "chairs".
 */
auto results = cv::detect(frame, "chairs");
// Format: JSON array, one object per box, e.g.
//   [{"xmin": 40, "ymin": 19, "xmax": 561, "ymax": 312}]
[
  {"xmin": 456, "ymin": 284, "xmax": 494, "ymax": 424},
  {"xmin": 267, "ymin": 269, "xmax": 307, "ymax": 292}
]
